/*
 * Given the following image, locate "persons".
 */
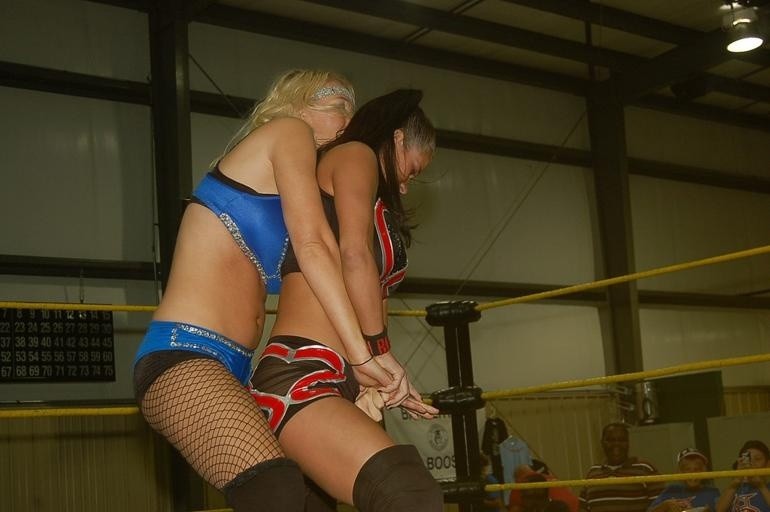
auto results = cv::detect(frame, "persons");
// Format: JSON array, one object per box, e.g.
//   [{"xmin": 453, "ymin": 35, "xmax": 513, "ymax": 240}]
[
  {"xmin": 715, "ymin": 440, "xmax": 770, "ymax": 511},
  {"xmin": 130, "ymin": 67, "xmax": 392, "ymax": 512},
  {"xmin": 475, "ymin": 451, "xmax": 507, "ymax": 511},
  {"xmin": 647, "ymin": 446, "xmax": 722, "ymax": 512},
  {"xmin": 579, "ymin": 421, "xmax": 665, "ymax": 512},
  {"xmin": 243, "ymin": 85, "xmax": 446, "ymax": 512},
  {"xmin": 508, "ymin": 462, "xmax": 580, "ymax": 511},
  {"xmin": 520, "ymin": 475, "xmax": 570, "ymax": 511}
]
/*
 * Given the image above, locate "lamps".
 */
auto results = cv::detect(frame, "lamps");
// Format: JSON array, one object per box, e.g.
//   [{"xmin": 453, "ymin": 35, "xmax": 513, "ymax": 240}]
[{"xmin": 717, "ymin": 1, "xmax": 769, "ymax": 58}]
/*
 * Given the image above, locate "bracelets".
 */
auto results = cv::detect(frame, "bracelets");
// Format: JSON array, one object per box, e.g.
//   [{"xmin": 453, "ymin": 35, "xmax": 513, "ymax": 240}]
[
  {"xmin": 348, "ymin": 353, "xmax": 373, "ymax": 368},
  {"xmin": 360, "ymin": 326, "xmax": 392, "ymax": 357}
]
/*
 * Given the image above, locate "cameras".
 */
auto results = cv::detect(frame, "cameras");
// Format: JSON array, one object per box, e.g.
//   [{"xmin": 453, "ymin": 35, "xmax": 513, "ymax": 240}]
[{"xmin": 740, "ymin": 449, "xmax": 750, "ymax": 468}]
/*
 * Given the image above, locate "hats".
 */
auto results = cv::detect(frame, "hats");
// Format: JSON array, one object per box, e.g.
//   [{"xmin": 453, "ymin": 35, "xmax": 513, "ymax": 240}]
[{"xmin": 676, "ymin": 446, "xmax": 709, "ymax": 465}]
